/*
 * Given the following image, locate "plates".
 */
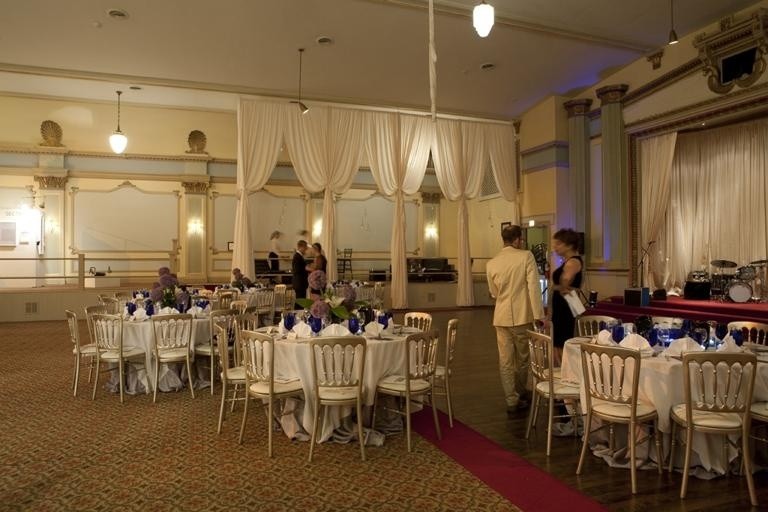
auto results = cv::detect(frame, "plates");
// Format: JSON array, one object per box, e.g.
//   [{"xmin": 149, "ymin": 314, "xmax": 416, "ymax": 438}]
[
  {"xmin": 746, "ymin": 344, "xmax": 768, "ymax": 353},
  {"xmin": 394, "ymin": 328, "xmax": 415, "ymax": 334},
  {"xmin": 255, "ymin": 326, "xmax": 279, "ymax": 333}
]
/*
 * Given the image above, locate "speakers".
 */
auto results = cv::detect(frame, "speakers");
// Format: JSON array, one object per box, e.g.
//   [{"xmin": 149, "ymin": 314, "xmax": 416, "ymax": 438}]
[
  {"xmin": 624, "ymin": 286, "xmax": 649, "ymax": 306},
  {"xmin": 684, "ymin": 282, "xmax": 711, "ymax": 300}
]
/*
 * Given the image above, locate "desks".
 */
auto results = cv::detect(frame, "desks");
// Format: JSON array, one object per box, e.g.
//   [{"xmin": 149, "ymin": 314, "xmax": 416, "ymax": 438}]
[{"xmin": 268, "ymin": 257, "xmax": 315, "ymax": 262}]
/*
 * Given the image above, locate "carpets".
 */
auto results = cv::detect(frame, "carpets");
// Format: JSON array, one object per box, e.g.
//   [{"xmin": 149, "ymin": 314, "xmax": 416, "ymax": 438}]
[
  {"xmin": 385, "ymin": 307, "xmax": 768, "ymax": 512},
  {"xmin": 0, "ymin": 318, "xmax": 524, "ymax": 512}
]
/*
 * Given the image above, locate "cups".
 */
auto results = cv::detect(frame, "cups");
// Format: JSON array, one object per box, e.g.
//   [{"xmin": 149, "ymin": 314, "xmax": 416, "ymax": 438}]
[
  {"xmin": 216, "ymin": 281, "xmax": 262, "ymax": 295},
  {"xmin": 132, "ymin": 289, "xmax": 150, "ymax": 299},
  {"xmin": 330, "ymin": 279, "xmax": 364, "ymax": 289},
  {"xmin": 192, "ymin": 289, "xmax": 198, "ymax": 296}
]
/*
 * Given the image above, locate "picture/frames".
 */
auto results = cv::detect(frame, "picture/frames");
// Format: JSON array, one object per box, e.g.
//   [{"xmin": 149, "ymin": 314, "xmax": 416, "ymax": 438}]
[
  {"xmin": 228, "ymin": 241, "xmax": 234, "ymax": 251},
  {"xmin": 0, "ymin": 220, "xmax": 18, "ymax": 246}
]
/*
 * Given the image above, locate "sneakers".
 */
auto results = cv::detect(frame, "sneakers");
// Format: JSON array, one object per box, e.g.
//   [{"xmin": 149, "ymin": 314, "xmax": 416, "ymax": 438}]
[{"xmin": 508, "ymin": 389, "xmax": 532, "ymax": 411}]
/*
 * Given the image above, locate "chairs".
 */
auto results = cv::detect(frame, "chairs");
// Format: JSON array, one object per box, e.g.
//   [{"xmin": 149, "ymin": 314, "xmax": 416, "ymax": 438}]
[
  {"xmin": 63, "ymin": 287, "xmax": 459, "ymax": 461},
  {"xmin": 577, "ymin": 315, "xmax": 618, "ymax": 337},
  {"xmin": 650, "ymin": 314, "xmax": 683, "ymax": 329},
  {"xmin": 669, "ymin": 351, "xmax": 760, "ymax": 506},
  {"xmin": 576, "ymin": 341, "xmax": 663, "ymax": 495},
  {"xmin": 337, "ymin": 248, "xmax": 353, "ymax": 273},
  {"xmin": 524, "ymin": 319, "xmax": 581, "ymax": 456},
  {"xmin": 727, "ymin": 321, "xmax": 768, "ymax": 345}
]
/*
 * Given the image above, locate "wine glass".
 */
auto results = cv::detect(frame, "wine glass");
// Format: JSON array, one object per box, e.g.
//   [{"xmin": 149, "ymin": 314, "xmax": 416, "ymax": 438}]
[
  {"xmin": 124, "ymin": 297, "xmax": 209, "ymax": 317},
  {"xmin": 594, "ymin": 319, "xmax": 745, "ymax": 354},
  {"xmin": 282, "ymin": 308, "xmax": 393, "ymax": 337}
]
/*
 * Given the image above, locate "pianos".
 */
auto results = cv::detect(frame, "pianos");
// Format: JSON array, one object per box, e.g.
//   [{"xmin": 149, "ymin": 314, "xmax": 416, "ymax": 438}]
[{"xmin": 389, "ymin": 258, "xmax": 455, "ymax": 282}]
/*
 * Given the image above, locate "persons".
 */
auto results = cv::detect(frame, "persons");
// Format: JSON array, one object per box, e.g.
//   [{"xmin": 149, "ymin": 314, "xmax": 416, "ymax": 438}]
[
  {"xmin": 309, "ymin": 242, "xmax": 327, "ymax": 299},
  {"xmin": 485, "ymin": 224, "xmax": 546, "ymax": 414},
  {"xmin": 548, "ymin": 227, "xmax": 585, "ymax": 368},
  {"xmin": 297, "ymin": 230, "xmax": 311, "ymax": 247},
  {"xmin": 291, "ymin": 239, "xmax": 309, "ymax": 310},
  {"xmin": 269, "ymin": 230, "xmax": 280, "ymax": 271}
]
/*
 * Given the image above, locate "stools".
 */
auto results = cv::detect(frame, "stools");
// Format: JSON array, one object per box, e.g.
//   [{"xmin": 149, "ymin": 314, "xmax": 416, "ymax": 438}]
[{"xmin": 368, "ymin": 268, "xmax": 387, "ymax": 282}]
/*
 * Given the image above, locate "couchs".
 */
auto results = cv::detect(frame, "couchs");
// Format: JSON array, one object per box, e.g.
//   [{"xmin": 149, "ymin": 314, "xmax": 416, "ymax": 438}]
[{"xmin": 406, "ymin": 256, "xmax": 448, "ymax": 272}]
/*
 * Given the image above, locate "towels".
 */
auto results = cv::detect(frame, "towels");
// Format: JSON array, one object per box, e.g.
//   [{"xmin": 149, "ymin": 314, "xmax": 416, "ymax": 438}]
[{"xmin": 590, "ymin": 330, "xmax": 745, "ymax": 357}]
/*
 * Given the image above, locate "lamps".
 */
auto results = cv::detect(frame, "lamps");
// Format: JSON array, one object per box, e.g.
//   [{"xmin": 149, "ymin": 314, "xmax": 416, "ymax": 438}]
[
  {"xmin": 290, "ymin": 49, "xmax": 310, "ymax": 114},
  {"xmin": 108, "ymin": 91, "xmax": 128, "ymax": 156},
  {"xmin": 472, "ymin": 1, "xmax": 495, "ymax": 38},
  {"xmin": 668, "ymin": 0, "xmax": 680, "ymax": 44}
]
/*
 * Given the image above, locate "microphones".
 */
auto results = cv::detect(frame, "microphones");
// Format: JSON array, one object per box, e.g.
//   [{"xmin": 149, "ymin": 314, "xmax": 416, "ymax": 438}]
[{"xmin": 648, "ymin": 240, "xmax": 656, "ymax": 244}]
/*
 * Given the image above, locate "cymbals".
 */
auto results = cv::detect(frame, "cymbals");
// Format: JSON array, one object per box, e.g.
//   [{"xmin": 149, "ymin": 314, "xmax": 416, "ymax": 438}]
[
  {"xmin": 750, "ymin": 260, "xmax": 768, "ymax": 264},
  {"xmin": 710, "ymin": 260, "xmax": 738, "ymax": 268}
]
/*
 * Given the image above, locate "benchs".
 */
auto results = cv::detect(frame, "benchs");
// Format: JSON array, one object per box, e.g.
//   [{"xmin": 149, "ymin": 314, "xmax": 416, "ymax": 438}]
[{"xmin": 389, "ymin": 269, "xmax": 455, "ymax": 283}]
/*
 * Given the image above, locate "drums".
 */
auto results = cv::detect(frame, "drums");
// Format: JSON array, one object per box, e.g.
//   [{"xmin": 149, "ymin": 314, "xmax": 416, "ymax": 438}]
[
  {"xmin": 723, "ymin": 279, "xmax": 753, "ymax": 303},
  {"xmin": 687, "ymin": 271, "xmax": 708, "ymax": 283},
  {"xmin": 711, "ymin": 275, "xmax": 733, "ymax": 290},
  {"xmin": 735, "ymin": 266, "xmax": 756, "ymax": 282}
]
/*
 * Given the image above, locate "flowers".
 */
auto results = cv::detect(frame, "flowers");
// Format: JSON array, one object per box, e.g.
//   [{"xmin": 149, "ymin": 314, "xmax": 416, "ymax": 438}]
[
  {"xmin": 296, "ymin": 269, "xmax": 370, "ymax": 325},
  {"xmin": 152, "ymin": 267, "xmax": 192, "ymax": 312}
]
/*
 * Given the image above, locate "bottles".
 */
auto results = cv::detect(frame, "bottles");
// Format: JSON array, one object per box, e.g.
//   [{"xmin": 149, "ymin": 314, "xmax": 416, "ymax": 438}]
[{"xmin": 589, "ymin": 291, "xmax": 598, "ymax": 309}]
